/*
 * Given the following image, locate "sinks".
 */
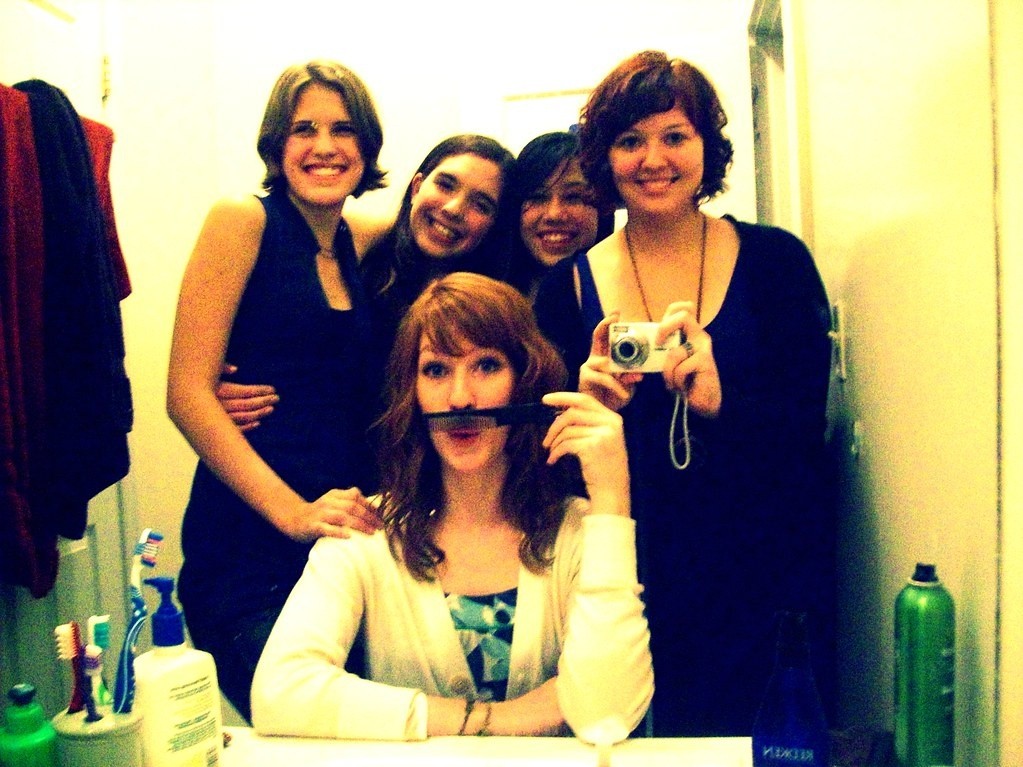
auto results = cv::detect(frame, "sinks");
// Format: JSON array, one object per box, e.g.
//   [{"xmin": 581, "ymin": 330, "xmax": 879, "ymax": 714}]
[{"xmin": 218, "ymin": 726, "xmax": 754, "ymax": 767}]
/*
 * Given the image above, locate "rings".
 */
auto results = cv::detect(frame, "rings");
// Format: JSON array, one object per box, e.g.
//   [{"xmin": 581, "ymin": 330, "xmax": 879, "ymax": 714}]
[{"xmin": 682, "ymin": 341, "xmax": 695, "ymax": 357}]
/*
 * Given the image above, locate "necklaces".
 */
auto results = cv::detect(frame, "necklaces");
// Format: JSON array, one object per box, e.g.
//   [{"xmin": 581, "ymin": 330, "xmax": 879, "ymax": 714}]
[{"xmin": 624, "ymin": 210, "xmax": 707, "ymax": 323}]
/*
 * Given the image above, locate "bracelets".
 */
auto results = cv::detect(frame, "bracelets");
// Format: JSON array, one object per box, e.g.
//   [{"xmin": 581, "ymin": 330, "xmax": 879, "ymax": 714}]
[{"xmin": 458, "ymin": 696, "xmax": 492, "ymax": 737}]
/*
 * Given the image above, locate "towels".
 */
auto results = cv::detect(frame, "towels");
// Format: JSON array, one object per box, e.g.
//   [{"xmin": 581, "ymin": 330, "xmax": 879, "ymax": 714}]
[{"xmin": 0, "ymin": 79, "xmax": 132, "ymax": 632}]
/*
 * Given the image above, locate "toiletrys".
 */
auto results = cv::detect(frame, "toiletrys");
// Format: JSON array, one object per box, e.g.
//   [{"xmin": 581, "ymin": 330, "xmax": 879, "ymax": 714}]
[
  {"xmin": 132, "ymin": 576, "xmax": 223, "ymax": 766},
  {"xmin": 1, "ymin": 684, "xmax": 55, "ymax": 766}
]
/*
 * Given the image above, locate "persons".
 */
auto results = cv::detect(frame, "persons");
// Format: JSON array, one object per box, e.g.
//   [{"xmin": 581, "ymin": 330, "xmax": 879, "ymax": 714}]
[
  {"xmin": 533, "ymin": 49, "xmax": 835, "ymax": 738},
  {"xmin": 167, "ymin": 64, "xmax": 392, "ymax": 722},
  {"xmin": 505, "ymin": 132, "xmax": 615, "ymax": 289},
  {"xmin": 215, "ymin": 135, "xmax": 519, "ymax": 435},
  {"xmin": 251, "ymin": 271, "xmax": 654, "ymax": 745}
]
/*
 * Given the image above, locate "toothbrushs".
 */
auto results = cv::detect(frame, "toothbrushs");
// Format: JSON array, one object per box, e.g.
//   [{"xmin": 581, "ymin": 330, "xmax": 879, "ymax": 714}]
[
  {"xmin": 114, "ymin": 528, "xmax": 165, "ymax": 713},
  {"xmin": 54, "ymin": 614, "xmax": 110, "ymax": 721}
]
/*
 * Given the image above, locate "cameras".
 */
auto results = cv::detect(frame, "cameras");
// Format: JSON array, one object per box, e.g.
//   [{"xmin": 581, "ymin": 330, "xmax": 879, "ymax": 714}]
[{"xmin": 607, "ymin": 322, "xmax": 682, "ymax": 373}]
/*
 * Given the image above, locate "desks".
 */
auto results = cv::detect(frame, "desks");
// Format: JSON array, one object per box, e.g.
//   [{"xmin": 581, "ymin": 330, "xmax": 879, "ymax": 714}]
[{"xmin": 222, "ymin": 725, "xmax": 752, "ymax": 767}]
